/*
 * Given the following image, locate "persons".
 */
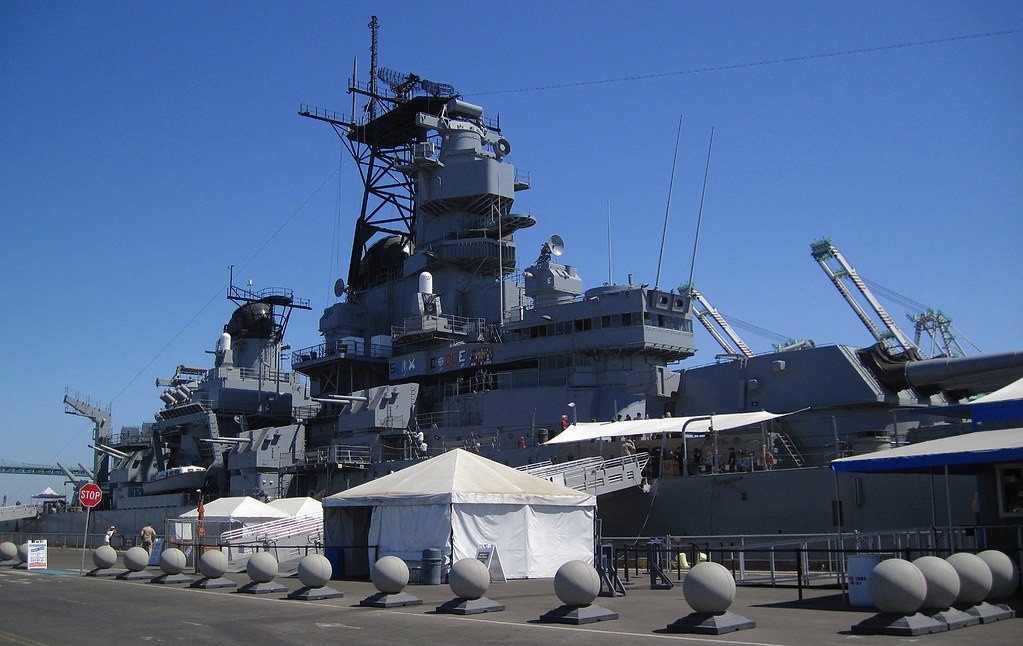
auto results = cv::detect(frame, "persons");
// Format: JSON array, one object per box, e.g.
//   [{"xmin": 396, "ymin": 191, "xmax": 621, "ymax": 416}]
[
  {"xmin": 413, "ymin": 430, "xmax": 428, "ymax": 458},
  {"xmin": 141, "ymin": 523, "xmax": 156, "ymax": 556},
  {"xmin": 106, "ymin": 526, "xmax": 117, "ymax": 545},
  {"xmin": 591, "ymin": 411, "xmax": 736, "ymax": 475},
  {"xmin": 44, "ymin": 499, "xmax": 67, "ymax": 513},
  {"xmin": 521, "ymin": 437, "xmax": 525, "ymax": 448},
  {"xmin": 561, "ymin": 414, "xmax": 568, "ymax": 431}
]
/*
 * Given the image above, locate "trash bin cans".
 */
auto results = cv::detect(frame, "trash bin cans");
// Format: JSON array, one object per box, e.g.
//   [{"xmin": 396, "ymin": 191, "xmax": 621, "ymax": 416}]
[{"xmin": 422, "ymin": 548, "xmax": 442, "ymax": 585}]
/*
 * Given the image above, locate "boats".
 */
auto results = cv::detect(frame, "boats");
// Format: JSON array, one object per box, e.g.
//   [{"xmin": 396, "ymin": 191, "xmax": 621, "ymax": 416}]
[
  {"xmin": 38, "ymin": 13, "xmax": 1023, "ymax": 564},
  {"xmin": 142, "ymin": 466, "xmax": 206, "ymax": 495}
]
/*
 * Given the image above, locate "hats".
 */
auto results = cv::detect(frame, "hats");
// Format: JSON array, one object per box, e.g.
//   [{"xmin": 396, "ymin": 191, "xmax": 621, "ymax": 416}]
[
  {"xmin": 637, "ymin": 413, "xmax": 642, "ymax": 415},
  {"xmin": 618, "ymin": 414, "xmax": 622, "ymax": 417},
  {"xmin": 729, "ymin": 447, "xmax": 734, "ymax": 450}
]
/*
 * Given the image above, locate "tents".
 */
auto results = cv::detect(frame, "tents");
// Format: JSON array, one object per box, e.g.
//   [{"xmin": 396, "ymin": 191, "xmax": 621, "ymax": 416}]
[
  {"xmin": 31, "ymin": 486, "xmax": 67, "ymax": 512},
  {"xmin": 322, "ymin": 448, "xmax": 597, "ymax": 584},
  {"xmin": 831, "ymin": 427, "xmax": 1023, "ymax": 607},
  {"xmin": 178, "ymin": 497, "xmax": 323, "ymax": 565}
]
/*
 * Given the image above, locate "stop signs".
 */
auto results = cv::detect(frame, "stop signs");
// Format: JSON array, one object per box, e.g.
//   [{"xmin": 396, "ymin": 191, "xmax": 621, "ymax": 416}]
[{"xmin": 78, "ymin": 483, "xmax": 102, "ymax": 508}]
